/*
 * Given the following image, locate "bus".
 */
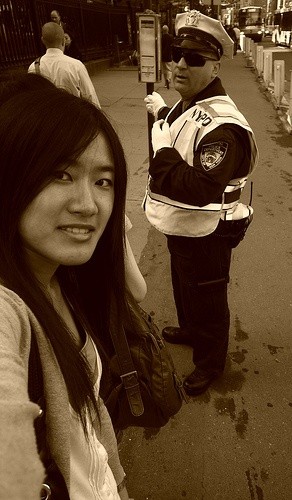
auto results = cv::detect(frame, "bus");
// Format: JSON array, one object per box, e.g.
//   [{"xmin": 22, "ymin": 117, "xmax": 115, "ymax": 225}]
[
  {"xmin": 238, "ymin": 6, "xmax": 264, "ymax": 42},
  {"xmin": 272, "ymin": 7, "xmax": 292, "ymax": 49}
]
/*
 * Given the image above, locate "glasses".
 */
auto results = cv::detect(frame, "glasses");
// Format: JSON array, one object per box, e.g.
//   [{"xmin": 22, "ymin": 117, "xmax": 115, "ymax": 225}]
[{"xmin": 170, "ymin": 44, "xmax": 217, "ymax": 67}]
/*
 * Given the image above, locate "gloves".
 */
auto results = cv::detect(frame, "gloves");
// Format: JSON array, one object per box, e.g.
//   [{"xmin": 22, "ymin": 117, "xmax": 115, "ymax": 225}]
[
  {"xmin": 144, "ymin": 92, "xmax": 166, "ymax": 120},
  {"xmin": 151, "ymin": 119, "xmax": 171, "ymax": 158}
]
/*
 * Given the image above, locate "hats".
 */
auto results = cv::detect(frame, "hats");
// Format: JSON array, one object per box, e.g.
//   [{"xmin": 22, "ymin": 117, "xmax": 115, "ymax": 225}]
[{"xmin": 175, "ymin": 10, "xmax": 233, "ymax": 60}]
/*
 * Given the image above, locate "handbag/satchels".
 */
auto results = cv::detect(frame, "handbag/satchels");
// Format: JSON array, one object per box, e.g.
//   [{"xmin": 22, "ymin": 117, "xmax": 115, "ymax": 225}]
[{"xmin": 85, "ymin": 284, "xmax": 186, "ymax": 429}]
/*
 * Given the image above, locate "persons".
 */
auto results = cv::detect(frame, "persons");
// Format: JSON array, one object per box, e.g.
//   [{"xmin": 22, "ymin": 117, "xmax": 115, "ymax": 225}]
[
  {"xmin": 26, "ymin": 21, "xmax": 103, "ymax": 111},
  {"xmin": 0, "ymin": 74, "xmax": 147, "ymax": 500},
  {"xmin": 50, "ymin": 10, "xmax": 83, "ymax": 63},
  {"xmin": 141, "ymin": 9, "xmax": 260, "ymax": 394},
  {"xmin": 160, "ymin": 25, "xmax": 172, "ymax": 87}
]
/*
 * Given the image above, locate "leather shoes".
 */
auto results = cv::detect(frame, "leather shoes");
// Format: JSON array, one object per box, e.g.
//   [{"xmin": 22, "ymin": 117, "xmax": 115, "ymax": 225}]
[
  {"xmin": 183, "ymin": 368, "xmax": 213, "ymax": 396},
  {"xmin": 162, "ymin": 326, "xmax": 191, "ymax": 344}
]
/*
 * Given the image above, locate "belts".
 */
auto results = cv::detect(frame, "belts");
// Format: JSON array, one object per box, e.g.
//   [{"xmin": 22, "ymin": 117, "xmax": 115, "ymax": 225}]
[{"xmin": 212, "ymin": 189, "xmax": 241, "ymax": 204}]
